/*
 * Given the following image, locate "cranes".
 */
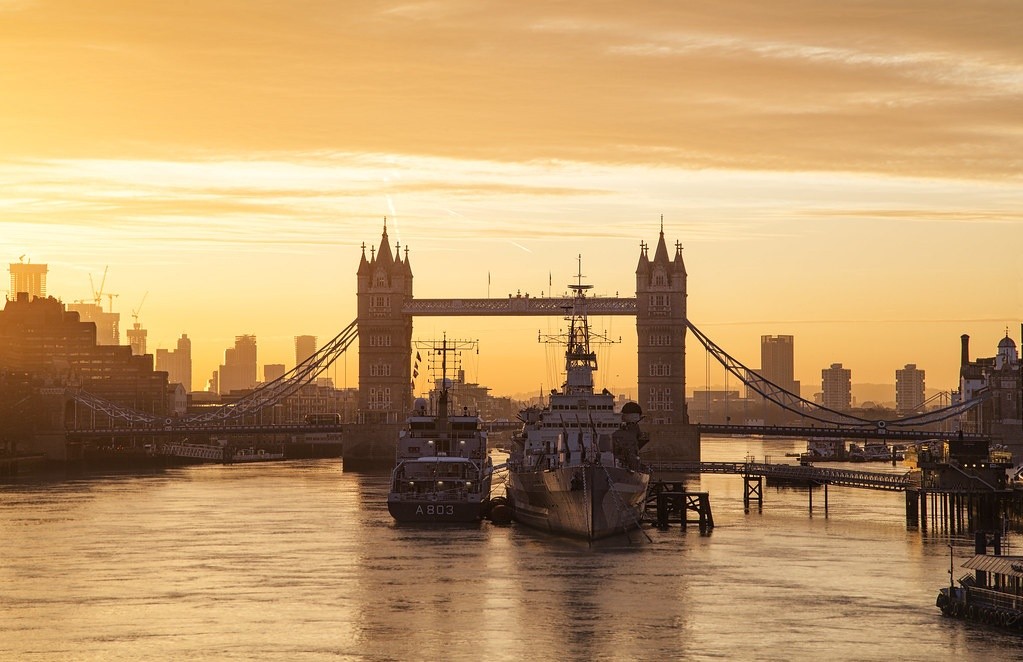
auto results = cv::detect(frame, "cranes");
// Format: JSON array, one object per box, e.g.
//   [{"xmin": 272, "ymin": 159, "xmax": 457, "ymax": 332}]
[
  {"xmin": 129, "ymin": 291, "xmax": 147, "ymax": 329},
  {"xmin": 97, "ymin": 291, "xmax": 119, "ymax": 313},
  {"xmin": 89, "ymin": 264, "xmax": 109, "ymax": 305}
]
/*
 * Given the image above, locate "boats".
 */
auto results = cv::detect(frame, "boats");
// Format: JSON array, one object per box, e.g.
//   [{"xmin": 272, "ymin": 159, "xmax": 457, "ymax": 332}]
[
  {"xmin": 935, "ymin": 543, "xmax": 1023, "ymax": 629},
  {"xmin": 387, "ymin": 330, "xmax": 494, "ymax": 524},
  {"xmin": 797, "ymin": 433, "xmax": 949, "ymax": 467},
  {"xmin": 231, "ymin": 447, "xmax": 287, "ymax": 463},
  {"xmin": 497, "ymin": 253, "xmax": 652, "ymax": 548}
]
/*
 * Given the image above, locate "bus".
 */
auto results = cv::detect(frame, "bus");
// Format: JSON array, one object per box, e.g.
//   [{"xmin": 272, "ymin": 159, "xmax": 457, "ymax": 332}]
[{"xmin": 304, "ymin": 413, "xmax": 342, "ymax": 426}]
